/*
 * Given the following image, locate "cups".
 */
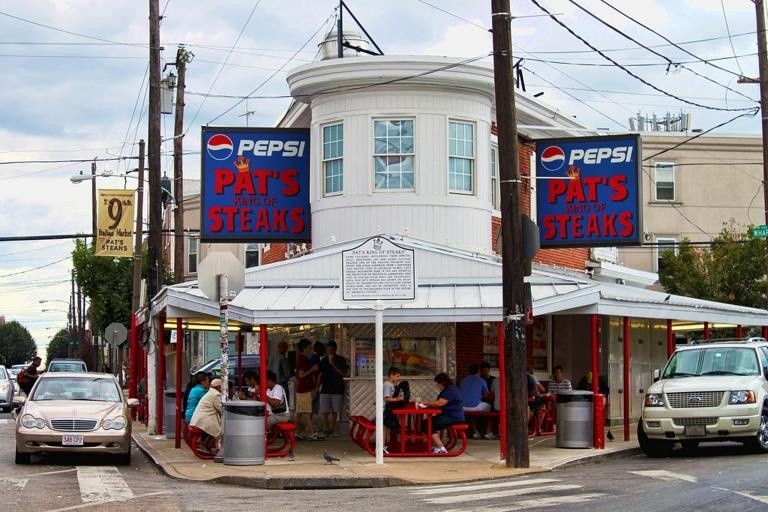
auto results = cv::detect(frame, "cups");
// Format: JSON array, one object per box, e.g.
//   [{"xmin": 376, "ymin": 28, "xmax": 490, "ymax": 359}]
[
  {"xmin": 329, "ymin": 353, "xmax": 333, "ymax": 361},
  {"xmin": 255, "ymin": 384, "xmax": 260, "ymax": 394},
  {"xmin": 414, "ymin": 397, "xmax": 420, "ymax": 408}
]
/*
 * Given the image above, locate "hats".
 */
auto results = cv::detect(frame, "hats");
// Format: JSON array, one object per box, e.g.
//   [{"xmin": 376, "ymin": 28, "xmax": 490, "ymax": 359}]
[{"xmin": 211, "ymin": 378, "xmax": 222, "ymax": 387}]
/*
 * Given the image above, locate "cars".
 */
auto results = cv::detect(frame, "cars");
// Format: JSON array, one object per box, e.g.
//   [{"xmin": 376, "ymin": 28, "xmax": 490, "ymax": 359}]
[
  {"xmin": 11, "ymin": 368, "xmax": 141, "ymax": 466},
  {"xmin": 0, "ymin": 362, "xmax": 47, "ymax": 413}
]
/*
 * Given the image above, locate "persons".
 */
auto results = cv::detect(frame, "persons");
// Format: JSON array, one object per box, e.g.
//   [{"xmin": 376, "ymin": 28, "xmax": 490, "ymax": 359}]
[
  {"xmin": 459, "ymin": 362, "xmax": 608, "ymax": 440},
  {"xmin": 419, "ymin": 372, "xmax": 464, "ymax": 453},
  {"xmin": 139, "ymin": 339, "xmax": 347, "ymax": 455},
  {"xmin": 22, "ymin": 356, "xmax": 42, "ymax": 396},
  {"xmin": 383, "ymin": 367, "xmax": 404, "ymax": 452}
]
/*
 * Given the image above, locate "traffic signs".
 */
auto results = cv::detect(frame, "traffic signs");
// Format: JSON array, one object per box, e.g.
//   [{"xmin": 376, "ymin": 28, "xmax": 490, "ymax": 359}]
[{"xmin": 750, "ymin": 226, "xmax": 768, "ymax": 238}]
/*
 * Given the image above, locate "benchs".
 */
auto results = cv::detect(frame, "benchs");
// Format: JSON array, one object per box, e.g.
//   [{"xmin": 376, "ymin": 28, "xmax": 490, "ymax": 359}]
[
  {"xmin": 345, "ymin": 395, "xmax": 556, "ymax": 458},
  {"xmin": 182, "ymin": 412, "xmax": 297, "ymax": 460}
]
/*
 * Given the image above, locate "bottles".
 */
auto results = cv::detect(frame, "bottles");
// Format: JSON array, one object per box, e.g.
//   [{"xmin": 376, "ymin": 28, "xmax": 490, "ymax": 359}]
[
  {"xmin": 235, "ymin": 390, "xmax": 239, "ymax": 399},
  {"xmin": 225, "ymin": 392, "xmax": 229, "ymax": 402},
  {"xmin": 398, "ymin": 388, "xmax": 405, "ymax": 396}
]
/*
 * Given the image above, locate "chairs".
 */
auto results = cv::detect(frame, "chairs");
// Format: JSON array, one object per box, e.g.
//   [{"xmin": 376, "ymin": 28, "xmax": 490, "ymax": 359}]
[
  {"xmin": 44, "ymin": 383, "xmax": 65, "ymax": 400},
  {"xmin": 88, "ymin": 384, "xmax": 111, "ymax": 401}
]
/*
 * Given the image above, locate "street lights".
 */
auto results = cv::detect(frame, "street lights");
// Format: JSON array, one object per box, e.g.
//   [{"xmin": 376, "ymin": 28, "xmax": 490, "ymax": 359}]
[
  {"xmin": 39, "ymin": 270, "xmax": 82, "ymax": 342},
  {"xmin": 69, "ymin": 169, "xmax": 184, "ymax": 449}
]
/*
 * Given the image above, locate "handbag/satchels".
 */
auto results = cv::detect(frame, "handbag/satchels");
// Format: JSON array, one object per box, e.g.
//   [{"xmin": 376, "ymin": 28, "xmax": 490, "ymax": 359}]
[
  {"xmin": 17, "ymin": 365, "xmax": 35, "ymax": 386},
  {"xmin": 272, "ymin": 401, "xmax": 286, "ymax": 413}
]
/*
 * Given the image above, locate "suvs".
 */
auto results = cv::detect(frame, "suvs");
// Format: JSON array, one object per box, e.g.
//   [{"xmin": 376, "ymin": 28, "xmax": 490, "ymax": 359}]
[
  {"xmin": 637, "ymin": 336, "xmax": 767, "ymax": 459},
  {"xmin": 188, "ymin": 353, "xmax": 262, "ymax": 388},
  {"xmin": 47, "ymin": 357, "xmax": 88, "ymax": 375}
]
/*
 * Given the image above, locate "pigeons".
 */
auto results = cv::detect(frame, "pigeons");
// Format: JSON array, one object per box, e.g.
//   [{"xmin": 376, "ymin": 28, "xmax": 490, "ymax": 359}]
[
  {"xmin": 664, "ymin": 295, "xmax": 670, "ymax": 301},
  {"xmin": 607, "ymin": 429, "xmax": 614, "ymax": 441},
  {"xmin": 324, "ymin": 450, "xmax": 340, "ymax": 465},
  {"xmin": 289, "ymin": 449, "xmax": 295, "ymax": 458}
]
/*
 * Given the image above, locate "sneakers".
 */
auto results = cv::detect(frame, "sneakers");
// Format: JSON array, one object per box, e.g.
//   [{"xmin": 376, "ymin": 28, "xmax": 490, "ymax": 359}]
[
  {"xmin": 294, "ymin": 430, "xmax": 339, "ymax": 441},
  {"xmin": 196, "ymin": 443, "xmax": 211, "ymax": 453},
  {"xmin": 474, "ymin": 431, "xmax": 498, "ymax": 440},
  {"xmin": 431, "ymin": 446, "xmax": 448, "ymax": 453}
]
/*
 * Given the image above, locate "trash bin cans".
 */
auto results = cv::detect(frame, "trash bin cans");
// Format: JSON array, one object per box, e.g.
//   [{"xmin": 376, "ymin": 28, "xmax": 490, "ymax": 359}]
[
  {"xmin": 556, "ymin": 389, "xmax": 594, "ymax": 448},
  {"xmin": 163, "ymin": 388, "xmax": 184, "ymax": 439},
  {"xmin": 222, "ymin": 399, "xmax": 265, "ymax": 465}
]
